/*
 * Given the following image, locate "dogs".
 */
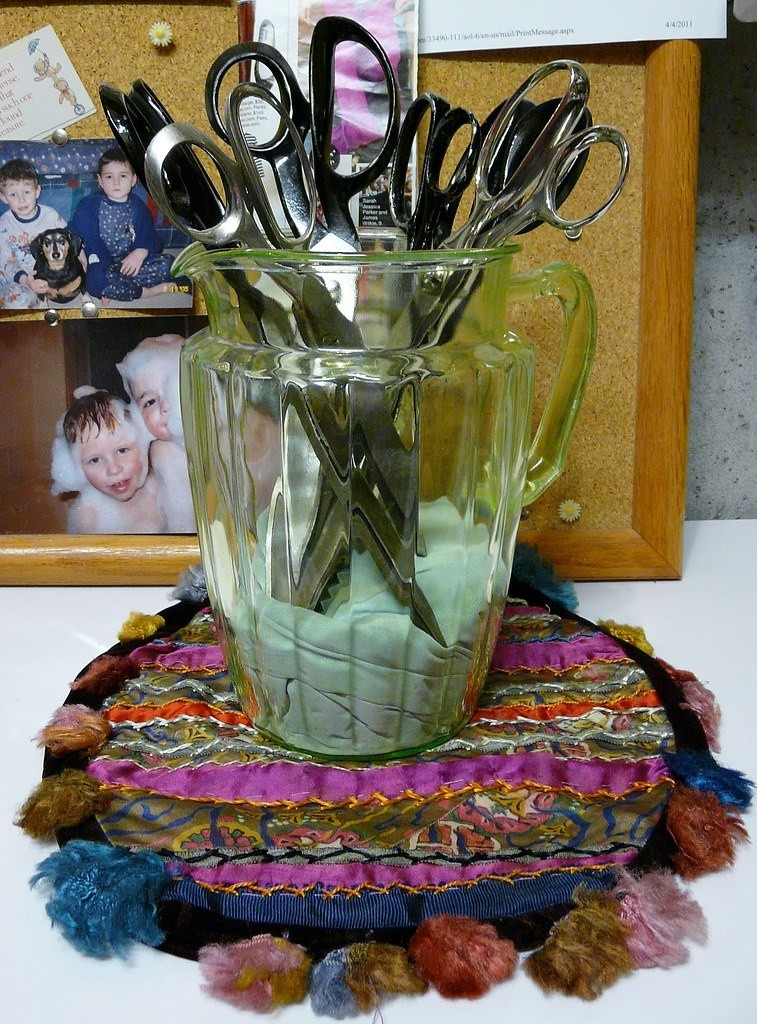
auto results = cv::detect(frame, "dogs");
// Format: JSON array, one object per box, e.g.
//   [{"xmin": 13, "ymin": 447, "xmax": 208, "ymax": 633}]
[{"xmin": 28, "ymin": 229, "xmax": 93, "ymax": 309}]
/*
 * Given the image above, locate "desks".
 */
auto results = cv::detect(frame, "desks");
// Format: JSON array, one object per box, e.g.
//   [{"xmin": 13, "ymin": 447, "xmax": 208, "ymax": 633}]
[{"xmin": 0, "ymin": 521, "xmax": 757, "ymax": 1024}]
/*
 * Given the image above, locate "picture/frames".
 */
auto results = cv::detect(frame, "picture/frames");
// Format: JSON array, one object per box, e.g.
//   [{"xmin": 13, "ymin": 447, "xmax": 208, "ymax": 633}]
[{"xmin": 0, "ymin": 0, "xmax": 701, "ymax": 584}]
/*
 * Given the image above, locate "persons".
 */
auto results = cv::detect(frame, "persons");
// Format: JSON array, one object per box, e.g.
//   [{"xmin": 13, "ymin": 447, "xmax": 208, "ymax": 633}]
[
  {"xmin": 351, "ymin": 151, "xmax": 412, "ymax": 206},
  {"xmin": 0, "ymin": 148, "xmax": 186, "ymax": 309},
  {"xmin": 52, "ymin": 332, "xmax": 281, "ymax": 535}
]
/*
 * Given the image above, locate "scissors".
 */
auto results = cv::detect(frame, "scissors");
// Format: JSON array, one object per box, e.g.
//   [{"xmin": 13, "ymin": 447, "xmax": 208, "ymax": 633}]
[{"xmin": 94, "ymin": 9, "xmax": 638, "ymax": 646}]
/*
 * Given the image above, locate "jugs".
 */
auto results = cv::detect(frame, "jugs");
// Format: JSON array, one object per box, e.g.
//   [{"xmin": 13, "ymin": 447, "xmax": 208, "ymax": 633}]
[{"xmin": 171, "ymin": 226, "xmax": 597, "ymax": 763}]
